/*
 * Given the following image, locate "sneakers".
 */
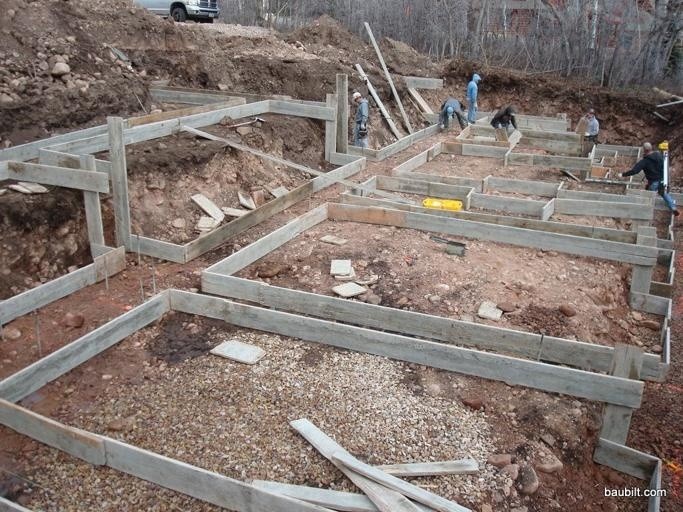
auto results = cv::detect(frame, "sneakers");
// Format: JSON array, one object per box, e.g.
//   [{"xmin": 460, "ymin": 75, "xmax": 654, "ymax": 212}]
[{"xmin": 671, "ymin": 207, "xmax": 680, "ymax": 217}]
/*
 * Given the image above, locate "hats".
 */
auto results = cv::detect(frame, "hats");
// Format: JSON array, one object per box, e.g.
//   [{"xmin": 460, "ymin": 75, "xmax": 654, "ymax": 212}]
[
  {"xmin": 588, "ymin": 108, "xmax": 595, "ymax": 114},
  {"xmin": 351, "ymin": 91, "xmax": 362, "ymax": 101},
  {"xmin": 446, "ymin": 106, "xmax": 454, "ymax": 117},
  {"xmin": 643, "ymin": 142, "xmax": 652, "ymax": 151},
  {"xmin": 507, "ymin": 104, "xmax": 517, "ymax": 117}
]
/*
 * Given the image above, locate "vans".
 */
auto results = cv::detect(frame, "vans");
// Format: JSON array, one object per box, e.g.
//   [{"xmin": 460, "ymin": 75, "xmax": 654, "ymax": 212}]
[{"xmin": 133, "ymin": 0, "xmax": 220, "ymax": 23}]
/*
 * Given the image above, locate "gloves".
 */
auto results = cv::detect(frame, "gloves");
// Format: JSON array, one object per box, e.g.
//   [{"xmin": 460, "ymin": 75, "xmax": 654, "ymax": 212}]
[
  {"xmin": 497, "ymin": 123, "xmax": 502, "ymax": 130},
  {"xmin": 359, "ymin": 123, "xmax": 365, "ymax": 130},
  {"xmin": 616, "ymin": 173, "xmax": 622, "ymax": 178},
  {"xmin": 584, "ymin": 131, "xmax": 590, "ymax": 136},
  {"xmin": 439, "ymin": 124, "xmax": 444, "ymax": 129},
  {"xmin": 472, "ymin": 102, "xmax": 477, "ymax": 107}
]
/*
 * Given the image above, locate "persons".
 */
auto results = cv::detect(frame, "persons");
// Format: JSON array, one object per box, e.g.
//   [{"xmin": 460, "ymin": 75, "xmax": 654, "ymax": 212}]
[
  {"xmin": 436, "ymin": 97, "xmax": 468, "ymax": 132},
  {"xmin": 465, "ymin": 74, "xmax": 481, "ymax": 126},
  {"xmin": 488, "ymin": 104, "xmax": 520, "ymax": 144},
  {"xmin": 573, "ymin": 108, "xmax": 599, "ymax": 147},
  {"xmin": 351, "ymin": 90, "xmax": 368, "ymax": 150},
  {"xmin": 616, "ymin": 141, "xmax": 681, "ymax": 218}
]
